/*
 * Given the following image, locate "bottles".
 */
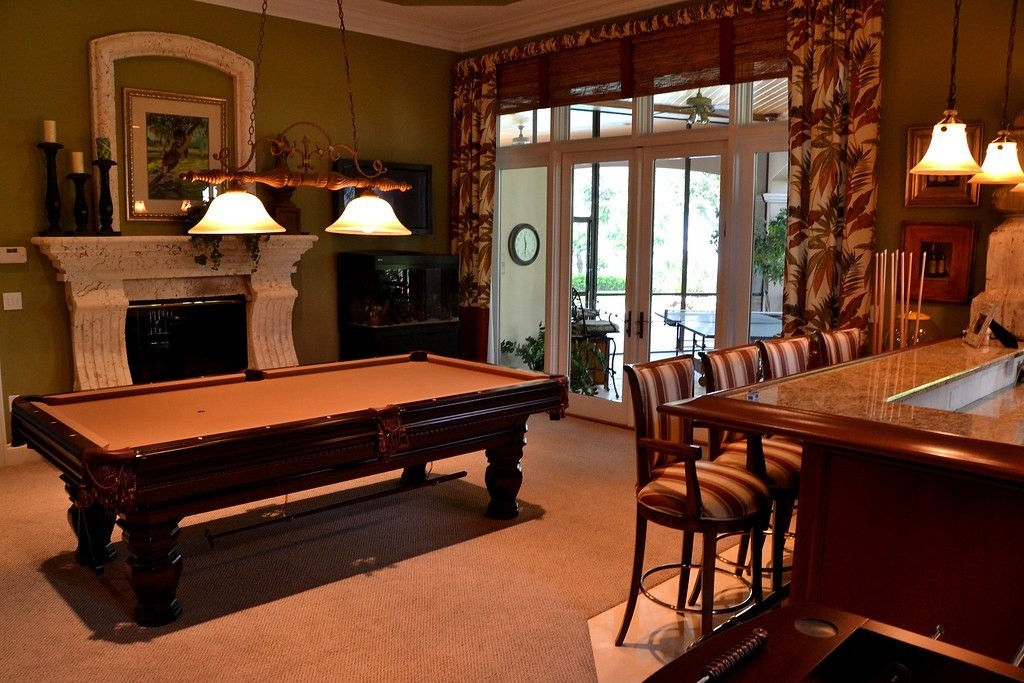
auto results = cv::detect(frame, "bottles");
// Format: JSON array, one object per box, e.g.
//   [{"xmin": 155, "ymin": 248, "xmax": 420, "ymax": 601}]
[{"xmin": 757, "ymin": 350, "xmax": 764, "ymax": 382}]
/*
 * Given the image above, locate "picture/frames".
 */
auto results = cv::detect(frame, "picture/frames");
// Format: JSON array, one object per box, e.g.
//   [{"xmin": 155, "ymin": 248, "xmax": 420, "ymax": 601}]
[
  {"xmin": 895, "ymin": 220, "xmax": 978, "ymax": 306},
  {"xmin": 120, "ymin": 86, "xmax": 229, "ymax": 223},
  {"xmin": 964, "ymin": 303, "xmax": 997, "ymax": 349},
  {"xmin": 334, "ymin": 158, "xmax": 434, "ymax": 238},
  {"xmin": 903, "ymin": 122, "xmax": 984, "ymax": 209}
]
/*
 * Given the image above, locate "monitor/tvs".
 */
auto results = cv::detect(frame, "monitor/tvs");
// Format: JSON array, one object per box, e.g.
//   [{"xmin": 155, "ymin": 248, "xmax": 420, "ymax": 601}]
[{"xmin": 333, "ymin": 159, "xmax": 433, "ymax": 236}]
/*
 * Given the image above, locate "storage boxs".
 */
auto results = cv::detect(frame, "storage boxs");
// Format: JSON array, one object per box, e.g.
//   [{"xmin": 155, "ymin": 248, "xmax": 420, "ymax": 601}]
[{"xmin": 336, "ymin": 249, "xmax": 460, "ymax": 328}]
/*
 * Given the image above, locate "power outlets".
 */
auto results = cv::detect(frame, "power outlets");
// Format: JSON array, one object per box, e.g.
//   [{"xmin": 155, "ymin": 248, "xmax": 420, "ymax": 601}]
[
  {"xmin": 8, "ymin": 395, "xmax": 21, "ymax": 413},
  {"xmin": 3, "ymin": 292, "xmax": 22, "ymax": 310}
]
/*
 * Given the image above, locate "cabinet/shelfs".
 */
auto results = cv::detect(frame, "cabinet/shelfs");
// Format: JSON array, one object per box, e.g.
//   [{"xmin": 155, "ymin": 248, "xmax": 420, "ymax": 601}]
[{"xmin": 336, "ymin": 317, "xmax": 462, "ymax": 361}]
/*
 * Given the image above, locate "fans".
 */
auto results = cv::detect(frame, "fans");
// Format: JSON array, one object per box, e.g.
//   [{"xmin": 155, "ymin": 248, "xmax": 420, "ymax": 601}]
[
  {"xmin": 511, "ymin": 125, "xmax": 531, "ymax": 145},
  {"xmin": 655, "ymin": 88, "xmax": 729, "ymax": 115}
]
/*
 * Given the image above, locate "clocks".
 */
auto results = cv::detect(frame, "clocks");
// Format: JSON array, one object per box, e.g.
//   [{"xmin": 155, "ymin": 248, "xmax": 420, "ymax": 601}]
[{"xmin": 508, "ymin": 223, "xmax": 540, "ymax": 266}]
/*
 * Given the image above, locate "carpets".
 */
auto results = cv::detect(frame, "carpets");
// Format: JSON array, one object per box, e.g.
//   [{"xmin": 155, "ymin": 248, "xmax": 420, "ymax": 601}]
[
  {"xmin": 0, "ymin": 410, "xmax": 741, "ymax": 683},
  {"xmin": 36, "ymin": 472, "xmax": 548, "ymax": 645}
]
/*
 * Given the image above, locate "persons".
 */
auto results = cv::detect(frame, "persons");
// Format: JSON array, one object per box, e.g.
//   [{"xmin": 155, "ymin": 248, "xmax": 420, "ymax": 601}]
[{"xmin": 156, "ymin": 128, "xmax": 192, "ymax": 183}]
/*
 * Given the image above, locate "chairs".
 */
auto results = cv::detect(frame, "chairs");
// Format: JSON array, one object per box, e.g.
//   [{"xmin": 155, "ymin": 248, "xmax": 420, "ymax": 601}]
[
  {"xmin": 699, "ymin": 343, "xmax": 797, "ymax": 579},
  {"xmin": 755, "ymin": 334, "xmax": 814, "ymax": 380},
  {"xmin": 615, "ymin": 355, "xmax": 773, "ymax": 649},
  {"xmin": 571, "ymin": 287, "xmax": 620, "ymax": 400},
  {"xmin": 820, "ymin": 326, "xmax": 862, "ymax": 368}
]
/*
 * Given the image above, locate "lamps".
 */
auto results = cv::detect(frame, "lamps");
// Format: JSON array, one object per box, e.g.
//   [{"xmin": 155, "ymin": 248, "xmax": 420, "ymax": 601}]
[
  {"xmin": 186, "ymin": 182, "xmax": 288, "ymax": 236},
  {"xmin": 685, "ymin": 109, "xmax": 710, "ymax": 125},
  {"xmin": 966, "ymin": 0, "xmax": 1024, "ymax": 185},
  {"xmin": 325, "ymin": 187, "xmax": 412, "ymax": 237},
  {"xmin": 909, "ymin": 0, "xmax": 983, "ymax": 178}
]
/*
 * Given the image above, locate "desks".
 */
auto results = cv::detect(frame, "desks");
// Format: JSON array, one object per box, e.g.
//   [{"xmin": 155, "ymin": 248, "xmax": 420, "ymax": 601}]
[
  {"xmin": 654, "ymin": 308, "xmax": 783, "ymax": 357},
  {"xmin": 11, "ymin": 351, "xmax": 570, "ymax": 628}
]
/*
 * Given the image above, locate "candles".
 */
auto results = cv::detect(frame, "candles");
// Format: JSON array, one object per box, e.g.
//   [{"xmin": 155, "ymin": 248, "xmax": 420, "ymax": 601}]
[
  {"xmin": 43, "ymin": 120, "xmax": 57, "ymax": 143},
  {"xmin": 97, "ymin": 138, "xmax": 112, "ymax": 160},
  {"xmin": 70, "ymin": 151, "xmax": 85, "ymax": 173}
]
